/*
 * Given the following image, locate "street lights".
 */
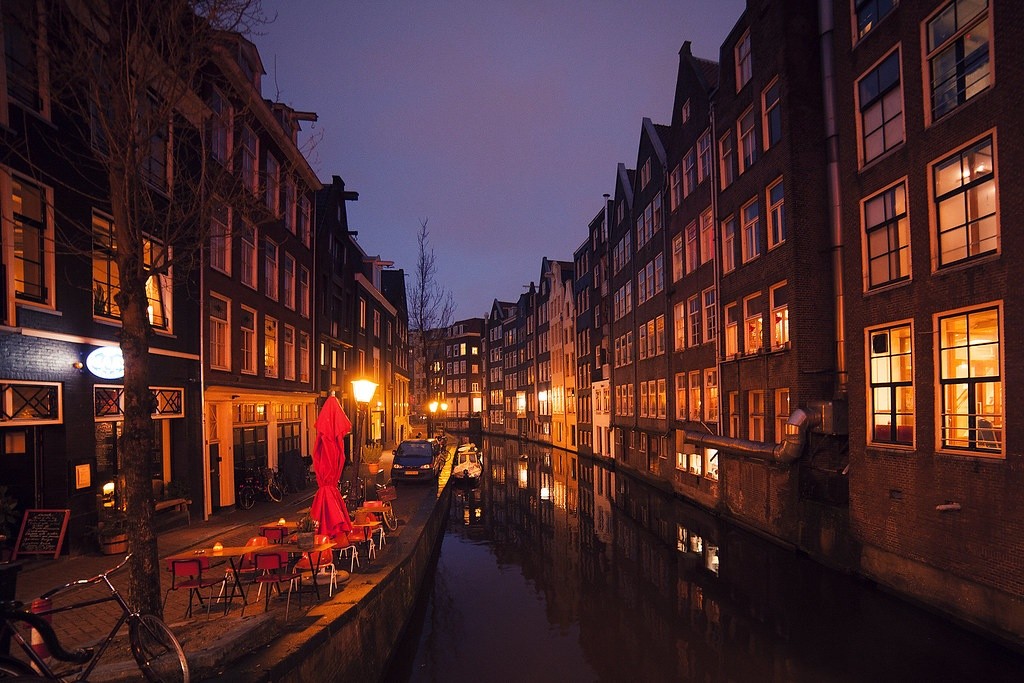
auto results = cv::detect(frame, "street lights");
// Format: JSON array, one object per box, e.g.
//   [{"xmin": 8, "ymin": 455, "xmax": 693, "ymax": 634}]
[
  {"xmin": 429, "ymin": 400, "xmax": 449, "ymax": 439},
  {"xmin": 347, "ymin": 377, "xmax": 379, "ymax": 510}
]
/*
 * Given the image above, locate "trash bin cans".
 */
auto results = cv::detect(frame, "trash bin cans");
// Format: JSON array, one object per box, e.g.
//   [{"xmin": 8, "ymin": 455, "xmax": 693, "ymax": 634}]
[{"xmin": 0, "ymin": 561, "xmax": 24, "ymax": 661}]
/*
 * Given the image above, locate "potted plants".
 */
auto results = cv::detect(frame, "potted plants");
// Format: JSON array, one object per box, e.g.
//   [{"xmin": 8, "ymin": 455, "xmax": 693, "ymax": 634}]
[
  {"xmin": 347, "ymin": 506, "xmax": 370, "ymax": 525},
  {"xmin": 296, "ymin": 511, "xmax": 315, "ymax": 547},
  {"xmin": 362, "ymin": 441, "xmax": 383, "ymax": 474},
  {"xmin": 91, "ymin": 505, "xmax": 130, "ymax": 553}
]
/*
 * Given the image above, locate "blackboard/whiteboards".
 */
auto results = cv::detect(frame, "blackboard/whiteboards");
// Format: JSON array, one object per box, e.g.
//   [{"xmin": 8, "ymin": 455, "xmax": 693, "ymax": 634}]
[{"xmin": 10, "ymin": 508, "xmax": 71, "ymax": 561}]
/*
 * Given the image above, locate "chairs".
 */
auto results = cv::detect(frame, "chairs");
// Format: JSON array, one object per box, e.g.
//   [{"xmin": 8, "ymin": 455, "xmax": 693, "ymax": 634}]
[{"xmin": 161, "ymin": 501, "xmax": 395, "ymax": 622}]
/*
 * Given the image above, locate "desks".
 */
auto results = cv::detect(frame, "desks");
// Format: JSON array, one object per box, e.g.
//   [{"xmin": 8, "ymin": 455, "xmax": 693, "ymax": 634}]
[
  {"xmin": 347, "ymin": 519, "xmax": 381, "ymax": 565},
  {"xmin": 250, "ymin": 542, "xmax": 336, "ymax": 608},
  {"xmin": 164, "ymin": 542, "xmax": 264, "ymax": 616},
  {"xmin": 297, "ymin": 506, "xmax": 311, "ymax": 513},
  {"xmin": 358, "ymin": 505, "xmax": 391, "ymax": 544}
]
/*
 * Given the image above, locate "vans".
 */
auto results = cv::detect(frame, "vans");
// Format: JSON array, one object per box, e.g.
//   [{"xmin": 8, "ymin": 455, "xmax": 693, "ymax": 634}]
[{"xmin": 390, "ymin": 438, "xmax": 441, "ymax": 484}]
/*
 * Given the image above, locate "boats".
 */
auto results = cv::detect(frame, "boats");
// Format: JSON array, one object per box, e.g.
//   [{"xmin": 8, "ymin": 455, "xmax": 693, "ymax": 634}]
[{"xmin": 453, "ymin": 445, "xmax": 485, "ymax": 480}]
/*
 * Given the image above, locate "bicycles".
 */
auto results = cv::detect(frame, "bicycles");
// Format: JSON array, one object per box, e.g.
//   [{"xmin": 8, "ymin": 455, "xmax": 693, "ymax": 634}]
[
  {"xmin": 335, "ymin": 477, "xmax": 366, "ymax": 511},
  {"xmin": 237, "ymin": 465, "xmax": 291, "ymax": 510},
  {"xmin": 440, "ymin": 446, "xmax": 451, "ymax": 462},
  {"xmin": 374, "ymin": 477, "xmax": 398, "ymax": 531},
  {"xmin": 1, "ymin": 552, "xmax": 191, "ymax": 683}
]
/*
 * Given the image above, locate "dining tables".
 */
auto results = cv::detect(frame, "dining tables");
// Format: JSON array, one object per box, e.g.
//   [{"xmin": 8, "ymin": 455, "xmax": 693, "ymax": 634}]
[{"xmin": 259, "ymin": 519, "xmax": 313, "ymax": 543}]
